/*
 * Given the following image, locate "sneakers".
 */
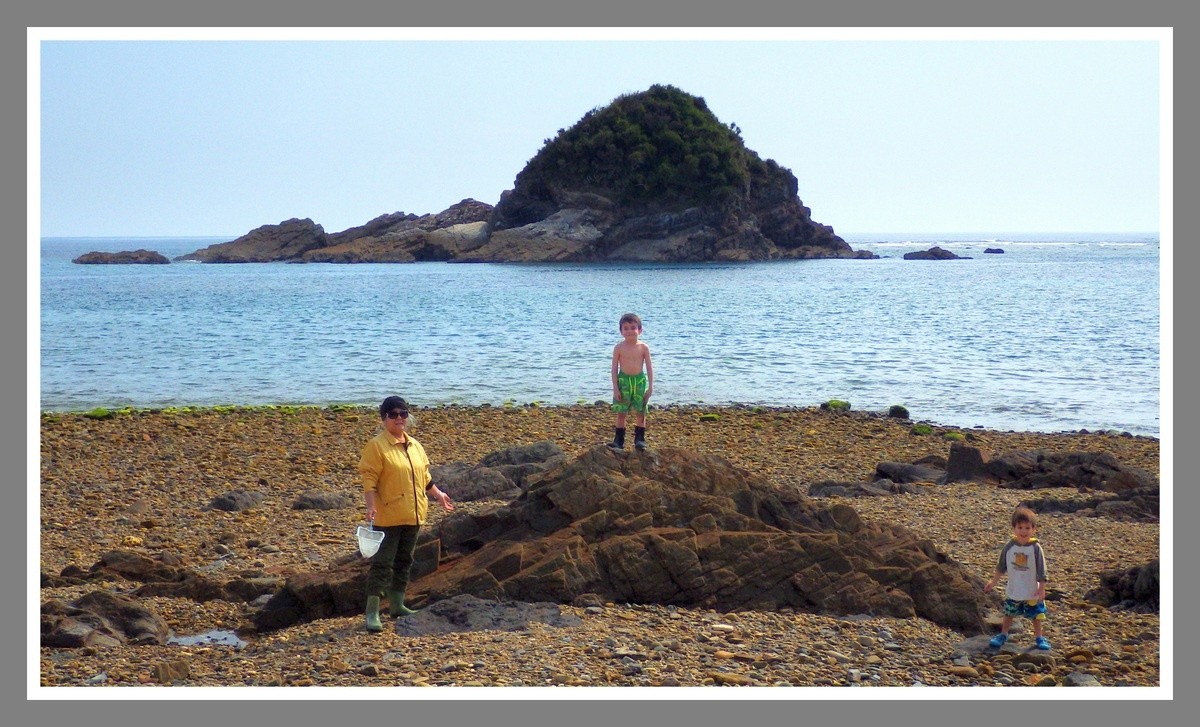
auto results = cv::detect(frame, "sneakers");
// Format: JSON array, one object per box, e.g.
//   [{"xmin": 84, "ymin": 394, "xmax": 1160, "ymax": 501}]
[
  {"xmin": 1036, "ymin": 636, "xmax": 1051, "ymax": 650},
  {"xmin": 989, "ymin": 633, "xmax": 1008, "ymax": 646}
]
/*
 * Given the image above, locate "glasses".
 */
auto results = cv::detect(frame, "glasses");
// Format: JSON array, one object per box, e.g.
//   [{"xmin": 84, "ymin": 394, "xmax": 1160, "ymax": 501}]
[{"xmin": 387, "ymin": 410, "xmax": 408, "ymax": 419}]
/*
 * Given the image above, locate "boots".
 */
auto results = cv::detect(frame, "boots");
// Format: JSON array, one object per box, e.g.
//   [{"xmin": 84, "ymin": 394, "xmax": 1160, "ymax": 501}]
[
  {"xmin": 634, "ymin": 425, "xmax": 648, "ymax": 450},
  {"xmin": 388, "ymin": 591, "xmax": 420, "ymax": 618},
  {"xmin": 607, "ymin": 426, "xmax": 626, "ymax": 450},
  {"xmin": 365, "ymin": 595, "xmax": 383, "ymax": 632}
]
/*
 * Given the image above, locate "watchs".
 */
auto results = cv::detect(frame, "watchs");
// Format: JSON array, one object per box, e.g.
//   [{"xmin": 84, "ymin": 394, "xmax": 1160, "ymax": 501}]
[{"xmin": 434, "ymin": 490, "xmax": 442, "ymax": 500}]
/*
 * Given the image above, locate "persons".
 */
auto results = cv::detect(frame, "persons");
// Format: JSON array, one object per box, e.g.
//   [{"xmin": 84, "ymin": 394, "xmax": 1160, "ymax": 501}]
[
  {"xmin": 983, "ymin": 505, "xmax": 1053, "ymax": 652},
  {"xmin": 605, "ymin": 312, "xmax": 655, "ymax": 450},
  {"xmin": 358, "ymin": 395, "xmax": 455, "ymax": 632}
]
